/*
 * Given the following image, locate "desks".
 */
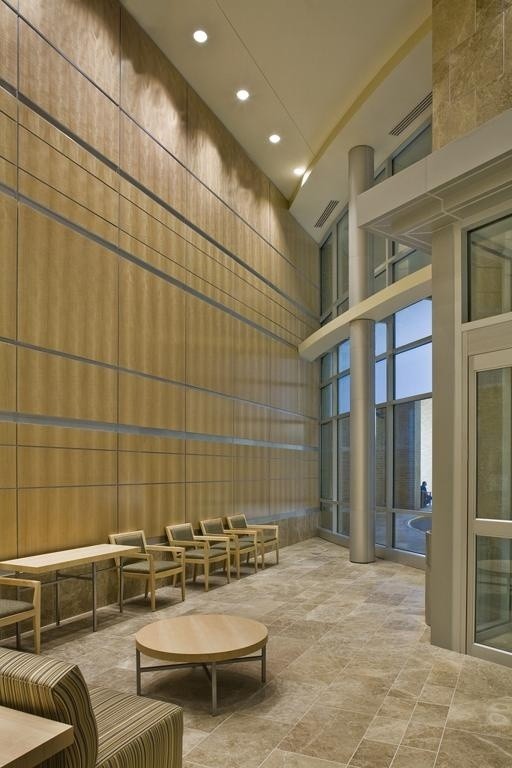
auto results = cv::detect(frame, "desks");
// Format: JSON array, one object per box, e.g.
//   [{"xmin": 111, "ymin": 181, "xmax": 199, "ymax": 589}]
[
  {"xmin": 0, "ymin": 544, "xmax": 142, "ymax": 651},
  {"xmin": 0, "ymin": 704, "xmax": 75, "ymax": 768}
]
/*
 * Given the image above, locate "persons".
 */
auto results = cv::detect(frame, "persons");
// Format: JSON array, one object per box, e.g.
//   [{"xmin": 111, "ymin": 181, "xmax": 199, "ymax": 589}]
[{"xmin": 419, "ymin": 480, "xmax": 433, "ymax": 508}]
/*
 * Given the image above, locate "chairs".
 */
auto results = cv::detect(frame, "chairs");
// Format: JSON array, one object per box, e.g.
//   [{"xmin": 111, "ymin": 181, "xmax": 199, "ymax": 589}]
[
  {"xmin": 107, "ymin": 512, "xmax": 281, "ymax": 611},
  {"xmin": 0, "ymin": 645, "xmax": 184, "ymax": 768},
  {"xmin": 0, "ymin": 578, "xmax": 41, "ymax": 657}
]
